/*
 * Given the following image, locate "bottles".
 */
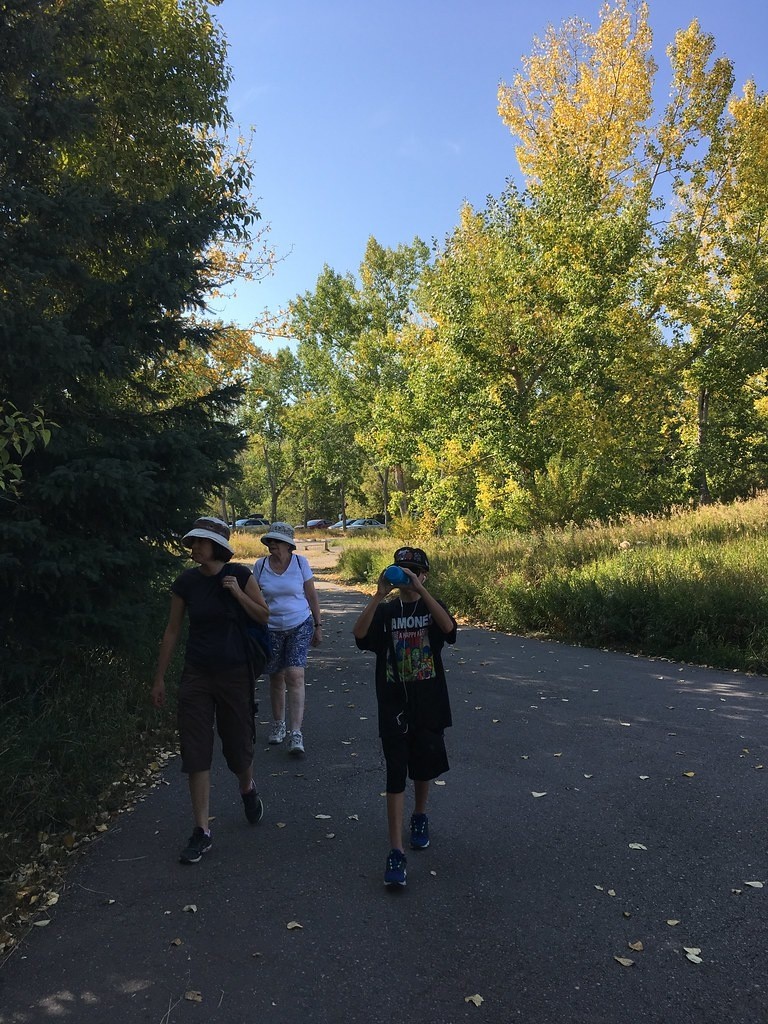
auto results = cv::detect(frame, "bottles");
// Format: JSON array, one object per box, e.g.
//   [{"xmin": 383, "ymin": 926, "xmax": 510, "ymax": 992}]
[{"xmin": 384, "ymin": 565, "xmax": 410, "ymax": 586}]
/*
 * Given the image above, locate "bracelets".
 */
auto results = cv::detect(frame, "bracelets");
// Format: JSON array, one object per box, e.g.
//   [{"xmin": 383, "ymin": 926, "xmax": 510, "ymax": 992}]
[{"xmin": 314, "ymin": 623, "xmax": 322, "ymax": 627}]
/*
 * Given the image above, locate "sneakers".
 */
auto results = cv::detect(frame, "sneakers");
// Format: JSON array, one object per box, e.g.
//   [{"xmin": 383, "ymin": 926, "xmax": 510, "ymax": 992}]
[
  {"xmin": 384, "ymin": 849, "xmax": 407, "ymax": 887},
  {"xmin": 287, "ymin": 730, "xmax": 305, "ymax": 754},
  {"xmin": 408, "ymin": 813, "xmax": 430, "ymax": 848},
  {"xmin": 240, "ymin": 778, "xmax": 264, "ymax": 824},
  {"xmin": 269, "ymin": 720, "xmax": 286, "ymax": 743}
]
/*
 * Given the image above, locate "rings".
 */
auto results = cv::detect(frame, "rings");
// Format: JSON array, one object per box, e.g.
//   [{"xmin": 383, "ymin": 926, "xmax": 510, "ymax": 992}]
[{"xmin": 226, "ymin": 581, "xmax": 229, "ymax": 584}]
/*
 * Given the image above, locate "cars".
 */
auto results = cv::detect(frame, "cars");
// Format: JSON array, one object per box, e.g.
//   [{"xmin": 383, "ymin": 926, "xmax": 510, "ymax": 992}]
[
  {"xmin": 293, "ymin": 520, "xmax": 335, "ymax": 530},
  {"xmin": 327, "ymin": 519, "xmax": 356, "ymax": 529},
  {"xmin": 339, "ymin": 519, "xmax": 385, "ymax": 530}
]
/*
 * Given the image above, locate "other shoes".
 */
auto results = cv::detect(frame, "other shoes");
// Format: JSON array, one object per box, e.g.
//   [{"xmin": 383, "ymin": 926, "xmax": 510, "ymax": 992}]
[{"xmin": 177, "ymin": 826, "xmax": 213, "ymax": 862}]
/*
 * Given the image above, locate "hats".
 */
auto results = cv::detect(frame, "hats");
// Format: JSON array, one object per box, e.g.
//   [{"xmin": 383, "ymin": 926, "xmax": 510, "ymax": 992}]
[
  {"xmin": 180, "ymin": 516, "xmax": 234, "ymax": 555},
  {"xmin": 261, "ymin": 522, "xmax": 297, "ymax": 550},
  {"xmin": 392, "ymin": 547, "xmax": 429, "ymax": 573}
]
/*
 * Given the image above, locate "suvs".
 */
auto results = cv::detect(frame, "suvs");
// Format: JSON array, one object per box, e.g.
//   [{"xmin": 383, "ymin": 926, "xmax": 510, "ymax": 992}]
[{"xmin": 229, "ymin": 519, "xmax": 271, "ymax": 528}]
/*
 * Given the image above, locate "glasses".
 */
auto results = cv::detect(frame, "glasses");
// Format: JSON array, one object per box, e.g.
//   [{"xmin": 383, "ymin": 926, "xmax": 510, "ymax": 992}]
[
  {"xmin": 400, "ymin": 565, "xmax": 427, "ymax": 577},
  {"xmin": 265, "ymin": 538, "xmax": 283, "ymax": 544}
]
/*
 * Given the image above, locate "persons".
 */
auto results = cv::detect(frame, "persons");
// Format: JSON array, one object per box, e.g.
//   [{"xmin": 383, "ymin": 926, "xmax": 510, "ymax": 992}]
[
  {"xmin": 351, "ymin": 546, "xmax": 457, "ymax": 888},
  {"xmin": 151, "ymin": 516, "xmax": 271, "ymax": 862},
  {"xmin": 252, "ymin": 522, "xmax": 323, "ymax": 752}
]
